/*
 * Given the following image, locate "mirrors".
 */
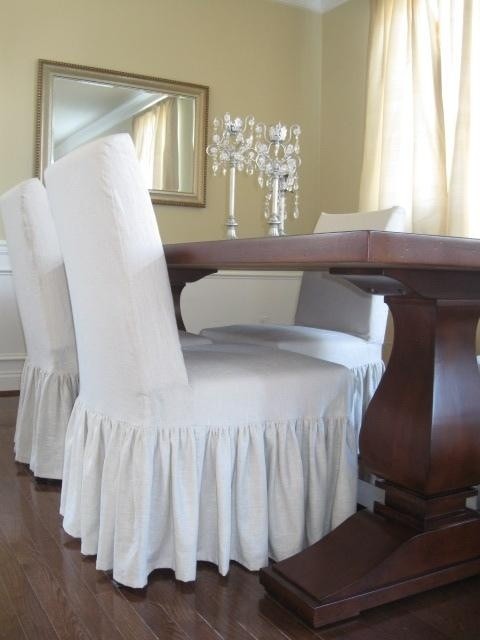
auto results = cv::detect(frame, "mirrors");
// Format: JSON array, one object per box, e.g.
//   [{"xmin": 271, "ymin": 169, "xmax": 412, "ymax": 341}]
[{"xmin": 35, "ymin": 59, "xmax": 209, "ymax": 207}]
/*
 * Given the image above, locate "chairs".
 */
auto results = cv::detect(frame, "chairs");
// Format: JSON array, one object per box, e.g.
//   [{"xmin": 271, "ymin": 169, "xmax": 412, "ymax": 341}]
[
  {"xmin": 0, "ymin": 178, "xmax": 212, "ymax": 479},
  {"xmin": 200, "ymin": 206, "xmax": 406, "ymax": 454},
  {"xmin": 43, "ymin": 133, "xmax": 359, "ymax": 587}
]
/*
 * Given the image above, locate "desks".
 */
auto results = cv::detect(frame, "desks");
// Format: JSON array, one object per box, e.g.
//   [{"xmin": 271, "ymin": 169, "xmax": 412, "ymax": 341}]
[{"xmin": 163, "ymin": 231, "xmax": 480, "ymax": 628}]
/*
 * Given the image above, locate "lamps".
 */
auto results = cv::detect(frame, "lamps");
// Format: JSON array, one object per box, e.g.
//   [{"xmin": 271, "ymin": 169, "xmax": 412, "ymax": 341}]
[
  {"xmin": 205, "ymin": 113, "xmax": 256, "ymax": 242},
  {"xmin": 252, "ymin": 122, "xmax": 302, "ymax": 236}
]
